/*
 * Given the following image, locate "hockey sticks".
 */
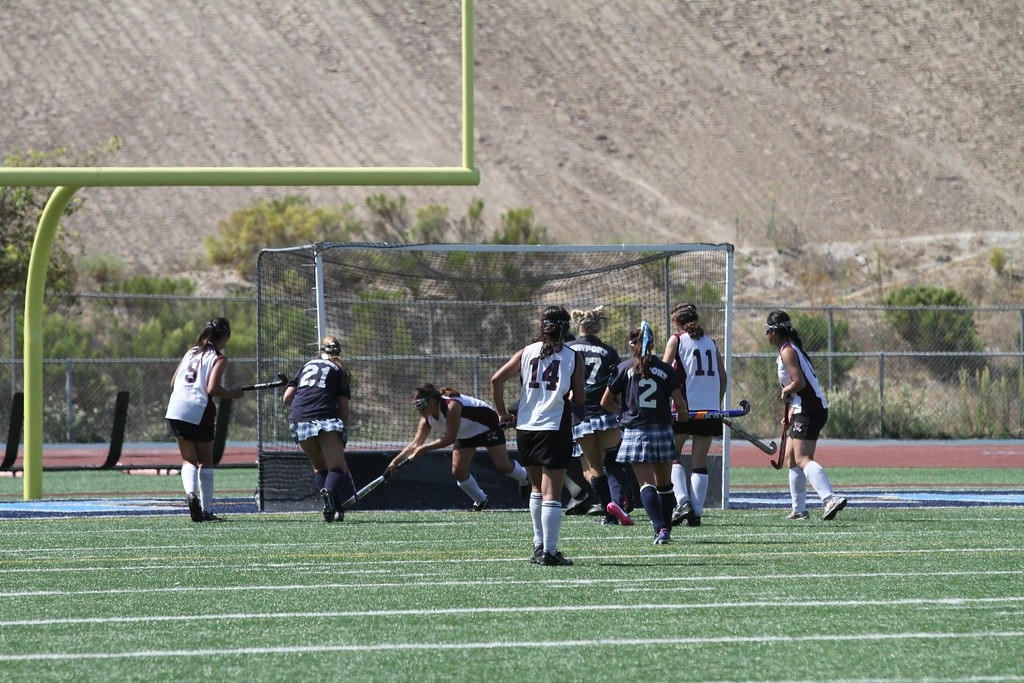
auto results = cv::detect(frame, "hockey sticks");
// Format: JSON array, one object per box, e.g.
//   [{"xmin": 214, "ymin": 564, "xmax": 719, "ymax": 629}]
[
  {"xmin": 616, "ymin": 399, "xmax": 751, "ymax": 422},
  {"xmin": 343, "ymin": 460, "xmax": 359, "ymax": 503},
  {"xmin": 341, "ymin": 452, "xmax": 415, "ymax": 511},
  {"xmin": 721, "ymin": 416, "xmax": 779, "ymax": 455},
  {"xmin": 499, "ymin": 363, "xmax": 619, "ymax": 431},
  {"xmin": 226, "ymin": 372, "xmax": 289, "ymax": 392},
  {"xmin": 770, "ymin": 393, "xmax": 792, "ymax": 470}
]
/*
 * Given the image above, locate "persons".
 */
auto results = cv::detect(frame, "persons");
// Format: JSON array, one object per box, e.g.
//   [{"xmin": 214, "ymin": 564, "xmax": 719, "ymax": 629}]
[
  {"xmin": 284, "ymin": 336, "xmax": 351, "ymax": 522},
  {"xmin": 663, "ymin": 302, "xmax": 726, "ymax": 526},
  {"xmin": 490, "ymin": 307, "xmax": 586, "ymax": 566},
  {"xmin": 388, "ymin": 384, "xmax": 530, "ymax": 511},
  {"xmin": 165, "ymin": 317, "xmax": 244, "ymax": 521},
  {"xmin": 765, "ymin": 310, "xmax": 847, "ymax": 521},
  {"xmin": 601, "ymin": 322, "xmax": 689, "ymax": 544},
  {"xmin": 563, "ymin": 306, "xmax": 635, "ymax": 527}
]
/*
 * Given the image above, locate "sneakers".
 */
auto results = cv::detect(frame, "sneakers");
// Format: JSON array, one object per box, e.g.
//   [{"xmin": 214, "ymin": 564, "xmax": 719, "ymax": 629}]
[
  {"xmin": 529, "ymin": 544, "xmax": 543, "ymax": 564},
  {"xmin": 564, "ymin": 492, "xmax": 589, "ymax": 515},
  {"xmin": 320, "ymin": 487, "xmax": 335, "ymax": 524},
  {"xmin": 518, "ymin": 465, "xmax": 529, "ymax": 498},
  {"xmin": 187, "ymin": 492, "xmax": 204, "ymax": 522},
  {"xmin": 606, "ymin": 501, "xmax": 635, "ymax": 525},
  {"xmin": 653, "ymin": 527, "xmax": 670, "ymax": 546},
  {"xmin": 334, "ymin": 510, "xmax": 344, "ymax": 521},
  {"xmin": 202, "ymin": 510, "xmax": 223, "ymax": 522},
  {"xmin": 785, "ymin": 510, "xmax": 810, "ymax": 521},
  {"xmin": 670, "ymin": 501, "xmax": 694, "ymax": 525},
  {"xmin": 821, "ymin": 496, "xmax": 847, "ymax": 520},
  {"xmin": 562, "ymin": 466, "xmax": 582, "ymax": 498},
  {"xmin": 540, "ymin": 550, "xmax": 573, "ymax": 566},
  {"xmin": 622, "ymin": 482, "xmax": 635, "ymax": 513},
  {"xmin": 586, "ymin": 501, "xmax": 606, "ymax": 515},
  {"xmin": 680, "ymin": 516, "xmax": 700, "ymax": 526},
  {"xmin": 472, "ymin": 494, "xmax": 489, "ymax": 513},
  {"xmin": 601, "ymin": 515, "xmax": 618, "ymax": 526}
]
